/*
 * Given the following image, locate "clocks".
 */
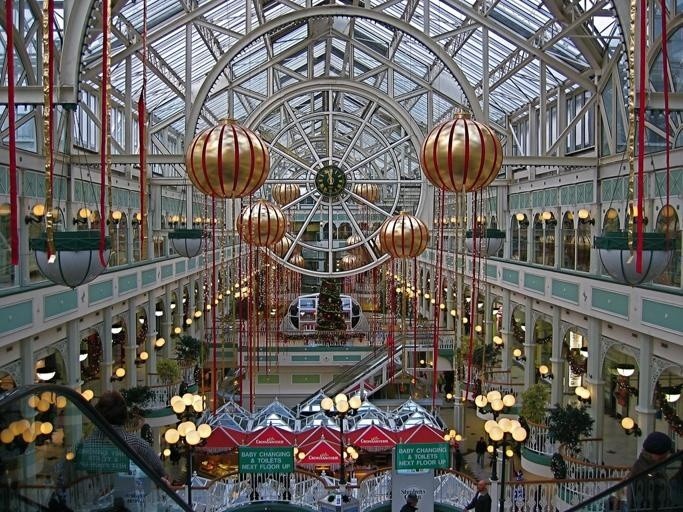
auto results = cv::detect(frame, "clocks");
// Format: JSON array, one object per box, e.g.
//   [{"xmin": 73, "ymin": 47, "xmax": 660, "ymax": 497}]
[{"xmin": 315, "ymin": 166, "xmax": 346, "ymax": 197}]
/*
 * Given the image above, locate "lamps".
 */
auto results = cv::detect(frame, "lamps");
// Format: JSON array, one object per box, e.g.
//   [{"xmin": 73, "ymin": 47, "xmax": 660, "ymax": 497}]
[
  {"xmin": 107, "ymin": 210, "xmax": 122, "ymax": 226},
  {"xmin": 73, "ymin": 206, "xmax": 90, "ymax": 226},
  {"xmin": 576, "ymin": 207, "xmax": 596, "ymax": 228},
  {"xmin": 516, "ymin": 212, "xmax": 530, "ymax": 227},
  {"xmin": 621, "ymin": 415, "xmax": 641, "ymax": 440},
  {"xmin": 24, "ymin": 203, "xmax": 46, "ymax": 226},
  {"xmin": 542, "ymin": 210, "xmax": 558, "ymax": 227}
]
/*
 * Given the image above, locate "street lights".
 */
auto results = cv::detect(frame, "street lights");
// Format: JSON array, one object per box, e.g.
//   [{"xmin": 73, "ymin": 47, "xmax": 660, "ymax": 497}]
[
  {"xmin": 443, "ymin": 391, "xmax": 527, "ymax": 512},
  {"xmin": 321, "ymin": 394, "xmax": 361, "ymax": 484},
  {"xmin": 161, "ymin": 393, "xmax": 213, "ymax": 507}
]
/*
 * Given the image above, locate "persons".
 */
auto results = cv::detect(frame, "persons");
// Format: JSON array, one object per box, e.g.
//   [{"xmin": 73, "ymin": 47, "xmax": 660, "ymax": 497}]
[
  {"xmin": 627, "ymin": 431, "xmax": 674, "ymax": 511},
  {"xmin": 475, "ymin": 437, "xmax": 487, "ymax": 468},
  {"xmin": 463, "ymin": 479, "xmax": 492, "ymax": 512},
  {"xmin": 400, "ymin": 494, "xmax": 418, "ymax": 512},
  {"xmin": 65, "ymin": 389, "xmax": 168, "ymax": 512},
  {"xmin": 455, "ymin": 449, "xmax": 462, "ymax": 477}
]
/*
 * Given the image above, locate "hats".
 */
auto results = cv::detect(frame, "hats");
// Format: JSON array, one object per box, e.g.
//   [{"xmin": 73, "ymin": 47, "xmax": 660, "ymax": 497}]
[{"xmin": 643, "ymin": 431, "xmax": 673, "ymax": 455}]
